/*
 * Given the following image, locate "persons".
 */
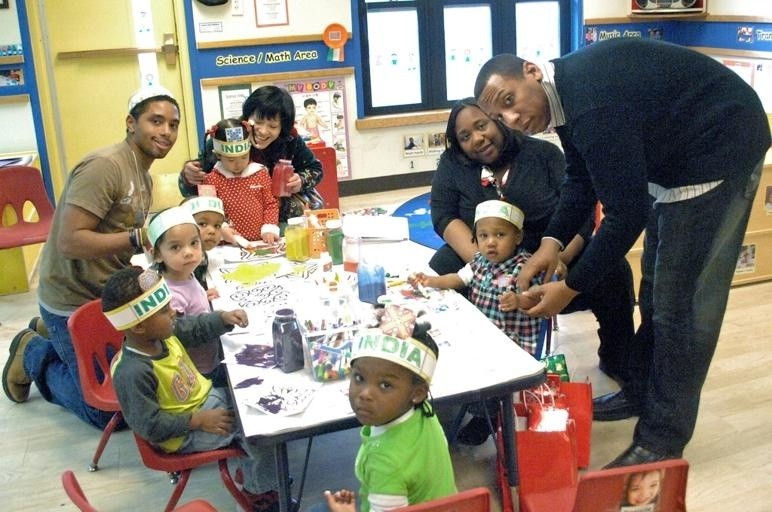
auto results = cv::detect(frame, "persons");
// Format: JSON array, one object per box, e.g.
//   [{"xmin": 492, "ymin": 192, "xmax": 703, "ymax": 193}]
[
  {"xmin": 178, "ymin": 193, "xmax": 226, "ymax": 293},
  {"xmin": 407, "ymin": 136, "xmax": 415, "ymax": 150},
  {"xmin": 100, "ymin": 264, "xmax": 301, "ymax": 509},
  {"xmin": 426, "ymin": 97, "xmax": 636, "ymax": 387},
  {"xmin": 323, "ymin": 317, "xmax": 460, "ymax": 510},
  {"xmin": 1, "ymin": 85, "xmax": 183, "ymax": 433},
  {"xmin": 177, "ymin": 84, "xmax": 325, "ymax": 223},
  {"xmin": 334, "ymin": 114, "xmax": 345, "ymax": 129},
  {"xmin": 333, "ymin": 92, "xmax": 341, "ymax": 105},
  {"xmin": 297, "ymin": 96, "xmax": 326, "ymax": 141},
  {"xmin": 471, "ymin": 35, "xmax": 771, "ymax": 468},
  {"xmin": 144, "ymin": 203, "xmax": 228, "ymax": 390},
  {"xmin": 433, "ymin": 135, "xmax": 446, "ymax": 147},
  {"xmin": 201, "ymin": 118, "xmax": 281, "ymax": 247},
  {"xmin": 412, "ymin": 195, "xmax": 552, "ymax": 445},
  {"xmin": 620, "ymin": 470, "xmax": 661, "ymax": 508}
]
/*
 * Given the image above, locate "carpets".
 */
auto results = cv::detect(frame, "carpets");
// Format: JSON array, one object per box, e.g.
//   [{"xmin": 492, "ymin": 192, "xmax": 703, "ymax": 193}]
[{"xmin": 391, "ymin": 191, "xmax": 447, "ymax": 250}]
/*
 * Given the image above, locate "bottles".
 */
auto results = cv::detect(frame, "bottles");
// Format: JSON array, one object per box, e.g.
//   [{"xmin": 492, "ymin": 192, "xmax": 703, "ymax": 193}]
[
  {"xmin": 273, "ymin": 309, "xmax": 304, "ymax": 374},
  {"xmin": 283, "ymin": 216, "xmax": 362, "ymax": 272},
  {"xmin": 0, "ymin": 45, "xmax": 22, "ymax": 86},
  {"xmin": 357, "ymin": 253, "xmax": 386, "ymax": 305},
  {"xmin": 270, "ymin": 157, "xmax": 294, "ymax": 199}
]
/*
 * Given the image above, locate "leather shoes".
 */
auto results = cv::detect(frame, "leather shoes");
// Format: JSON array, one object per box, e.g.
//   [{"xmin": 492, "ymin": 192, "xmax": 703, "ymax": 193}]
[
  {"xmin": 593, "ymin": 389, "xmax": 639, "ymax": 420},
  {"xmin": 603, "ymin": 444, "xmax": 682, "ymax": 469}
]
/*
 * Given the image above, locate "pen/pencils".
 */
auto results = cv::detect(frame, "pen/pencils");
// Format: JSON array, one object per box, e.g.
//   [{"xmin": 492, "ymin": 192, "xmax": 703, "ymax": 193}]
[
  {"xmin": 305, "ymin": 319, "xmax": 361, "ymax": 379},
  {"xmin": 411, "ymin": 275, "xmax": 431, "ymax": 299}
]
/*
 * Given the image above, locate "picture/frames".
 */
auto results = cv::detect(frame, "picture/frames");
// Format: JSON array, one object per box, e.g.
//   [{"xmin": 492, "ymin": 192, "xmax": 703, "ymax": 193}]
[{"xmin": 253, "ymin": 0, "xmax": 290, "ymax": 29}]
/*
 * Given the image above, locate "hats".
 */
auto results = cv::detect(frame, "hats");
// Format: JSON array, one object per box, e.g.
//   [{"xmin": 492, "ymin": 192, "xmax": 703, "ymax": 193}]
[{"xmin": 130, "ymin": 86, "xmax": 174, "ymax": 113}]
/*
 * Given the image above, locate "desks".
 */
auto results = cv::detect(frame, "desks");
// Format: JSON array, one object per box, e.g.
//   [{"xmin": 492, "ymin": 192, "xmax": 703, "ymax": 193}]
[{"xmin": 201, "ymin": 238, "xmax": 549, "ymax": 512}]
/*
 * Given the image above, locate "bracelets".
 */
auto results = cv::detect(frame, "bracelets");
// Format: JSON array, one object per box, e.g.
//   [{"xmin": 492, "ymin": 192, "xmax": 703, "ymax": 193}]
[{"xmin": 126, "ymin": 227, "xmax": 145, "ymax": 250}]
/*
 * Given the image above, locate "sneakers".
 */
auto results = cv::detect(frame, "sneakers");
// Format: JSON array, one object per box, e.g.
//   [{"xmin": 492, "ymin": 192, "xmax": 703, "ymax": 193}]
[
  {"xmin": 2, "ymin": 317, "xmax": 50, "ymax": 403},
  {"xmin": 459, "ymin": 416, "xmax": 497, "ymax": 446}
]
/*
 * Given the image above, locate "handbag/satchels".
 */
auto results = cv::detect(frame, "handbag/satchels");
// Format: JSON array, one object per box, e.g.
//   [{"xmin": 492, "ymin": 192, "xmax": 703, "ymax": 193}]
[{"xmin": 500, "ymin": 372, "xmax": 592, "ymax": 490}]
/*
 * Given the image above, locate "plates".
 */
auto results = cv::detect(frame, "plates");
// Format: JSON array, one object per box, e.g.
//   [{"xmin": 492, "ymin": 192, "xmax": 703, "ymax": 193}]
[{"xmin": 323, "ymin": 23, "xmax": 349, "ymax": 48}]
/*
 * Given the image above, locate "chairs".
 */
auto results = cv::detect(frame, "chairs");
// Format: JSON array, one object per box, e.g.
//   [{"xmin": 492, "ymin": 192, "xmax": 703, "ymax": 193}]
[{"xmin": 0, "ymin": 165, "xmax": 54, "ymax": 251}]
